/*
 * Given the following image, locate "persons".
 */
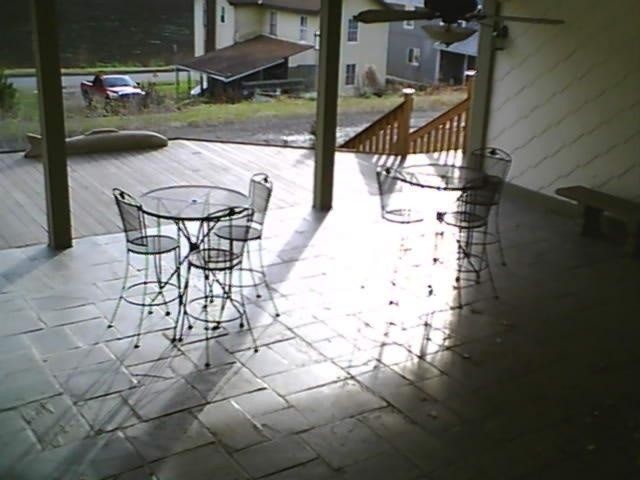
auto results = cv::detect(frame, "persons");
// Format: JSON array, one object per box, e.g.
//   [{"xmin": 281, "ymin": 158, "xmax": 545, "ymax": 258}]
[{"xmin": 91, "ymin": 70, "xmax": 105, "ymax": 88}]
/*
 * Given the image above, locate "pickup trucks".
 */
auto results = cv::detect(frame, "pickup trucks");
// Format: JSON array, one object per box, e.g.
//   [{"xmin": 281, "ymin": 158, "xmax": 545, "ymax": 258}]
[{"xmin": 80, "ymin": 73, "xmax": 148, "ymax": 110}]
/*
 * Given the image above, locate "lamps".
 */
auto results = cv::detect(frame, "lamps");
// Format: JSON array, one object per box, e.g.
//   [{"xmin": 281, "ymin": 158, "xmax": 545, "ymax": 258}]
[
  {"xmin": 489, "ymin": 25, "xmax": 509, "ymax": 50},
  {"xmin": 420, "ymin": 18, "xmax": 479, "ymax": 47}
]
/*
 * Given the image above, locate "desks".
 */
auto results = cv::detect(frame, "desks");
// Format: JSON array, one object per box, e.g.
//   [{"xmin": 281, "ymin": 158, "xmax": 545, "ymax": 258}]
[
  {"xmin": 136, "ymin": 184, "xmax": 253, "ymax": 330},
  {"xmin": 394, "ymin": 162, "xmax": 486, "ymax": 248}
]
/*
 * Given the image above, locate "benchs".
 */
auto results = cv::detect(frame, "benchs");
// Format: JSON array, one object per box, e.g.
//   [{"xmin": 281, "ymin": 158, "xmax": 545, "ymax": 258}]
[{"xmin": 554, "ymin": 184, "xmax": 640, "ymax": 257}]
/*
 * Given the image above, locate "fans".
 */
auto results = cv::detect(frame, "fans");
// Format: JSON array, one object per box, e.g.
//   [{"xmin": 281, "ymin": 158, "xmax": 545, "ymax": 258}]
[{"xmin": 352, "ymin": 1, "xmax": 565, "ymax": 25}]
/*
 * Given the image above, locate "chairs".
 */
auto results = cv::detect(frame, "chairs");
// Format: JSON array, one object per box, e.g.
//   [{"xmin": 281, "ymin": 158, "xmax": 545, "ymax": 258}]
[
  {"xmin": 107, "ymin": 188, "xmax": 196, "ymax": 349},
  {"xmin": 211, "ymin": 173, "xmax": 280, "ymax": 329},
  {"xmin": 376, "ymin": 166, "xmax": 426, "ymax": 264},
  {"xmin": 452, "ymin": 147, "xmax": 511, "ymax": 266},
  {"xmin": 429, "ymin": 175, "xmax": 501, "ymax": 308},
  {"xmin": 175, "ymin": 207, "xmax": 259, "ymax": 369}
]
